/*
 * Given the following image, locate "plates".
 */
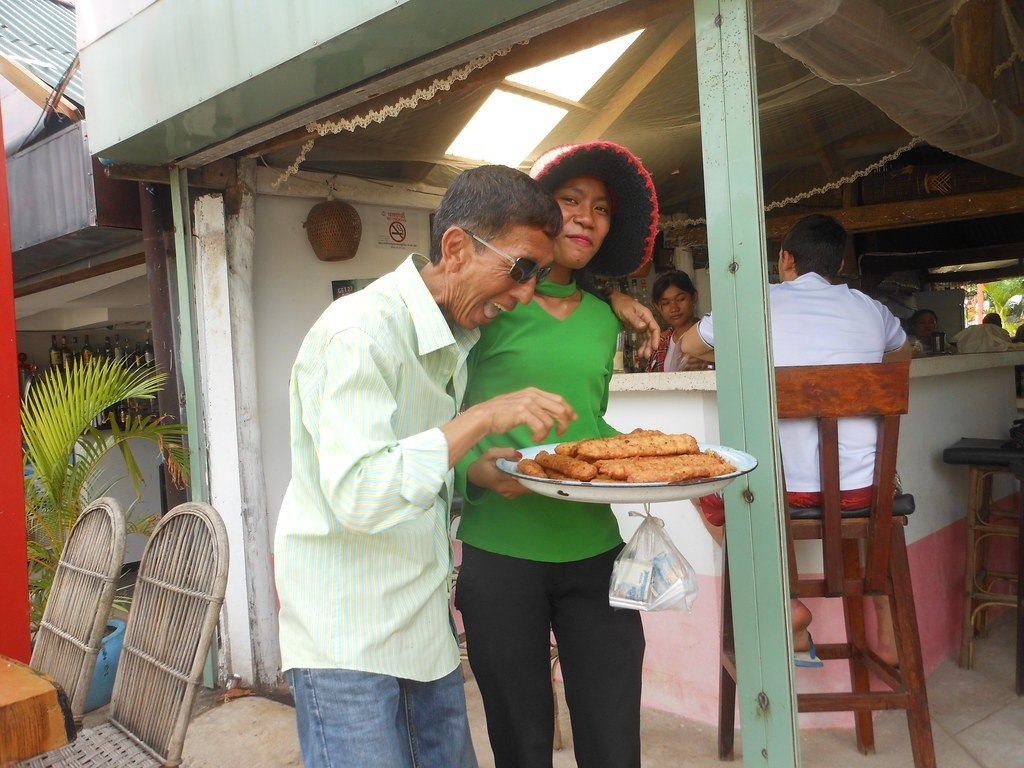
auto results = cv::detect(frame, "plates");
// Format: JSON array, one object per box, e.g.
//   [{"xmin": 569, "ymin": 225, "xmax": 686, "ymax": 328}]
[
  {"xmin": 925, "ymin": 350, "xmax": 952, "ymax": 355},
  {"xmin": 495, "ymin": 441, "xmax": 758, "ymax": 503}
]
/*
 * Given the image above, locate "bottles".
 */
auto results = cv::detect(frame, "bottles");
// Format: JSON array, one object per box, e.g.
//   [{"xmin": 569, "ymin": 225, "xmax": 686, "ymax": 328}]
[
  {"xmin": 949, "ymin": 341, "xmax": 958, "ymax": 355},
  {"xmin": 590, "ymin": 276, "xmax": 661, "ymax": 374},
  {"xmin": 16, "ymin": 331, "xmax": 158, "ymax": 433}
]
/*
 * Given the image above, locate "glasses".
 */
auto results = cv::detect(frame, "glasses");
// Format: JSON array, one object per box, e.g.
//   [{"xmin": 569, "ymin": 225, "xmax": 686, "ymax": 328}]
[{"xmin": 441, "ymin": 229, "xmax": 551, "ymax": 284}]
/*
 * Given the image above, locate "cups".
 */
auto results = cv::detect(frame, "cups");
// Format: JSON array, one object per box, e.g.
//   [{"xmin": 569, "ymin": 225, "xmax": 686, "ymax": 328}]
[{"xmin": 930, "ymin": 332, "xmax": 947, "ymax": 351}]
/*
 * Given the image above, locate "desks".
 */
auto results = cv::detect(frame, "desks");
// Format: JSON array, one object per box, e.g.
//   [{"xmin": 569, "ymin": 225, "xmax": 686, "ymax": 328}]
[{"xmin": 1, "ymin": 655, "xmax": 77, "ymax": 768}]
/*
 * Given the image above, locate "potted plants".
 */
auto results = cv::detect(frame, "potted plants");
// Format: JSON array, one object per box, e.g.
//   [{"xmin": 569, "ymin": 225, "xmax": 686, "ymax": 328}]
[{"xmin": 18, "ymin": 350, "xmax": 193, "ymax": 713}]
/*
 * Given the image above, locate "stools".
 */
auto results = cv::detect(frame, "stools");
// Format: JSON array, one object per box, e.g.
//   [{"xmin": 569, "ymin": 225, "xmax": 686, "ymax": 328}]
[
  {"xmin": 717, "ymin": 361, "xmax": 937, "ymax": 768},
  {"xmin": 944, "ymin": 437, "xmax": 1024, "ymax": 669}
]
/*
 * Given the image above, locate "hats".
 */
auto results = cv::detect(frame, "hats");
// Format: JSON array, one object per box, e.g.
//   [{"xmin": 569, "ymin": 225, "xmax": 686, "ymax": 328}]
[{"xmin": 529, "ymin": 141, "xmax": 658, "ymax": 278}]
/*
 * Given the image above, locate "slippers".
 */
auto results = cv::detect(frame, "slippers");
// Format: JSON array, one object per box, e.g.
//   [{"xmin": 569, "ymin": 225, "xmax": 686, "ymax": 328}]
[{"xmin": 792, "ymin": 632, "xmax": 825, "ymax": 666}]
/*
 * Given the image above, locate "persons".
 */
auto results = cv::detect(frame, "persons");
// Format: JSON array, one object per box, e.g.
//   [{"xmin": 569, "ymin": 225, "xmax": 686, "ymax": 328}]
[
  {"xmin": 446, "ymin": 142, "xmax": 656, "ymax": 768},
  {"xmin": 646, "ymin": 267, "xmax": 708, "ymax": 371},
  {"xmin": 903, "ymin": 306, "xmax": 939, "ymax": 358},
  {"xmin": 681, "ymin": 214, "xmax": 913, "ymax": 663},
  {"xmin": 272, "ymin": 164, "xmax": 661, "ymax": 768},
  {"xmin": 983, "ymin": 313, "xmax": 1024, "ymax": 397}
]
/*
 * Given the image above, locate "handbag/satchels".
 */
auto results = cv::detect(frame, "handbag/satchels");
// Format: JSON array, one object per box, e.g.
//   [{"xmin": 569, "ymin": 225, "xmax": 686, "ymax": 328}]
[{"xmin": 606, "ymin": 503, "xmax": 698, "ymax": 610}]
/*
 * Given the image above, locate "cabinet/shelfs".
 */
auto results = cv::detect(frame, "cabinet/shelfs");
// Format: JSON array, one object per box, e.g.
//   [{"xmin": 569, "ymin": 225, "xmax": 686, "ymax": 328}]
[{"xmin": 33, "ymin": 365, "xmax": 156, "ymax": 429}]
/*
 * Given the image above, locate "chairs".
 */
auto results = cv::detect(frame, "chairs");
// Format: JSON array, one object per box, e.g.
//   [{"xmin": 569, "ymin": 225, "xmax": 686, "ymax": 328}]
[
  {"xmin": 7, "ymin": 501, "xmax": 230, "ymax": 768},
  {"xmin": 27, "ymin": 496, "xmax": 127, "ymax": 730}
]
